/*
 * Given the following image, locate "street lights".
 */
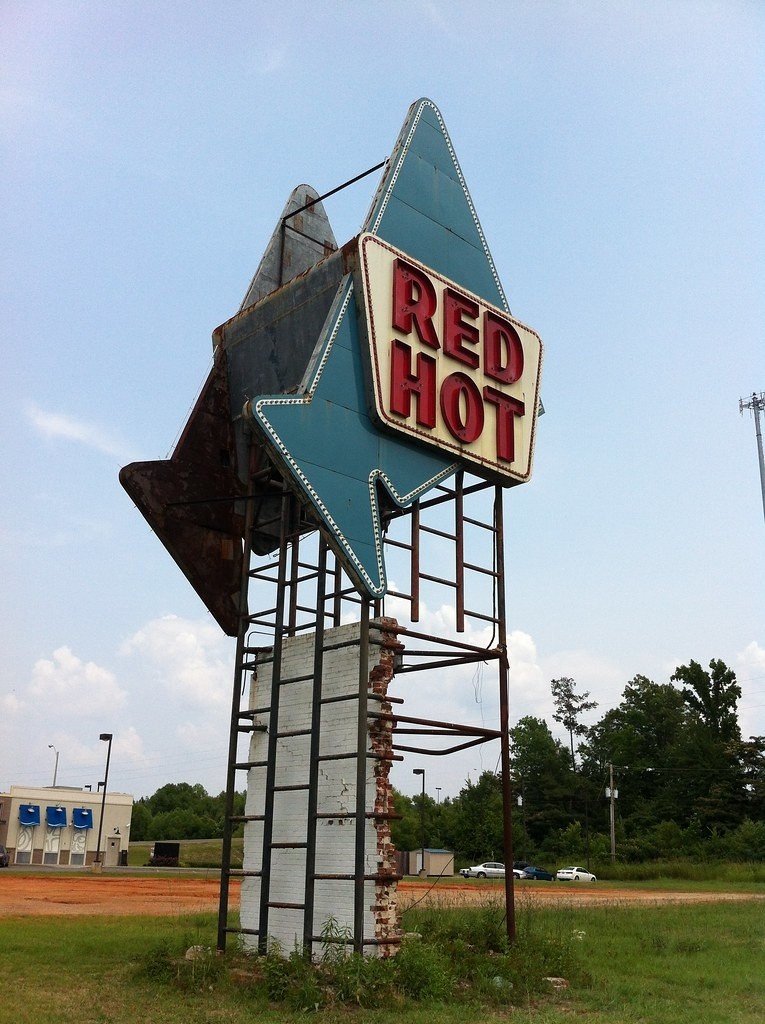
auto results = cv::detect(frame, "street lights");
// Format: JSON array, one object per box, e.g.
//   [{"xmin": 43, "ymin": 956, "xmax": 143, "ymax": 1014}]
[
  {"xmin": 92, "ymin": 734, "xmax": 112, "ymax": 874},
  {"xmin": 436, "ymin": 788, "xmax": 441, "ymax": 804},
  {"xmin": 97, "ymin": 782, "xmax": 105, "ymax": 792},
  {"xmin": 85, "ymin": 785, "xmax": 91, "ymax": 792},
  {"xmin": 413, "ymin": 769, "xmax": 428, "ymax": 879},
  {"xmin": 48, "ymin": 744, "xmax": 58, "ymax": 787}
]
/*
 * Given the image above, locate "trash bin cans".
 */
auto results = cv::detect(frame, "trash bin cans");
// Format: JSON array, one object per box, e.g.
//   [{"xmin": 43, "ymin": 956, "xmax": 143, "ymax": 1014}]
[{"xmin": 122, "ymin": 850, "xmax": 127, "ymax": 866}]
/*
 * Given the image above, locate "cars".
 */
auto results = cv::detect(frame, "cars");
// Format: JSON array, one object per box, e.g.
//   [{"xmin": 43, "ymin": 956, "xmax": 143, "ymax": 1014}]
[
  {"xmin": 0, "ymin": 844, "xmax": 12, "ymax": 867},
  {"xmin": 513, "ymin": 861, "xmax": 530, "ymax": 870},
  {"xmin": 459, "ymin": 862, "xmax": 527, "ymax": 879},
  {"xmin": 523, "ymin": 866, "xmax": 556, "ymax": 881},
  {"xmin": 557, "ymin": 866, "xmax": 596, "ymax": 882}
]
